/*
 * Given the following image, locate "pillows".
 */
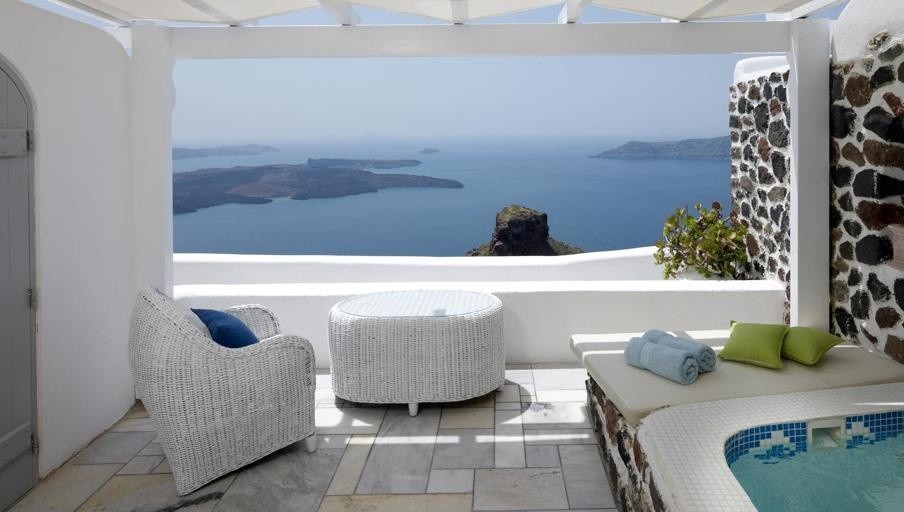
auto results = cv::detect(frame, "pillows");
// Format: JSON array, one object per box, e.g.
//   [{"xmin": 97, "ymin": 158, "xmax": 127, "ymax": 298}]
[
  {"xmin": 191, "ymin": 302, "xmax": 261, "ymax": 346},
  {"xmin": 720, "ymin": 319, "xmax": 845, "ymax": 369}
]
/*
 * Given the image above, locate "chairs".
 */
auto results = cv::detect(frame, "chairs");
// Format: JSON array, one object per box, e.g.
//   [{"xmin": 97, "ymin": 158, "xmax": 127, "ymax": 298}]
[{"xmin": 135, "ymin": 284, "xmax": 320, "ymax": 499}]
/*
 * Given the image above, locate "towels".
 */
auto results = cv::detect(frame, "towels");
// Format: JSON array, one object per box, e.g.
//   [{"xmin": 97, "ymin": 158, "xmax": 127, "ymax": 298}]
[{"xmin": 627, "ymin": 318, "xmax": 714, "ymax": 387}]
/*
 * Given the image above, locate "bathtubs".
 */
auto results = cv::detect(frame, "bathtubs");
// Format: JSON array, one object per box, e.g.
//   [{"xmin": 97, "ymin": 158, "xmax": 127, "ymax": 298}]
[{"xmin": 719, "ymin": 398, "xmax": 904, "ymax": 511}]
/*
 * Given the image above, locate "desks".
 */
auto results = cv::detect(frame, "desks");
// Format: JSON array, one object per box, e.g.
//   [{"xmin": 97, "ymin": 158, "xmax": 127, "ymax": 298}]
[{"xmin": 330, "ymin": 291, "xmax": 507, "ymax": 414}]
